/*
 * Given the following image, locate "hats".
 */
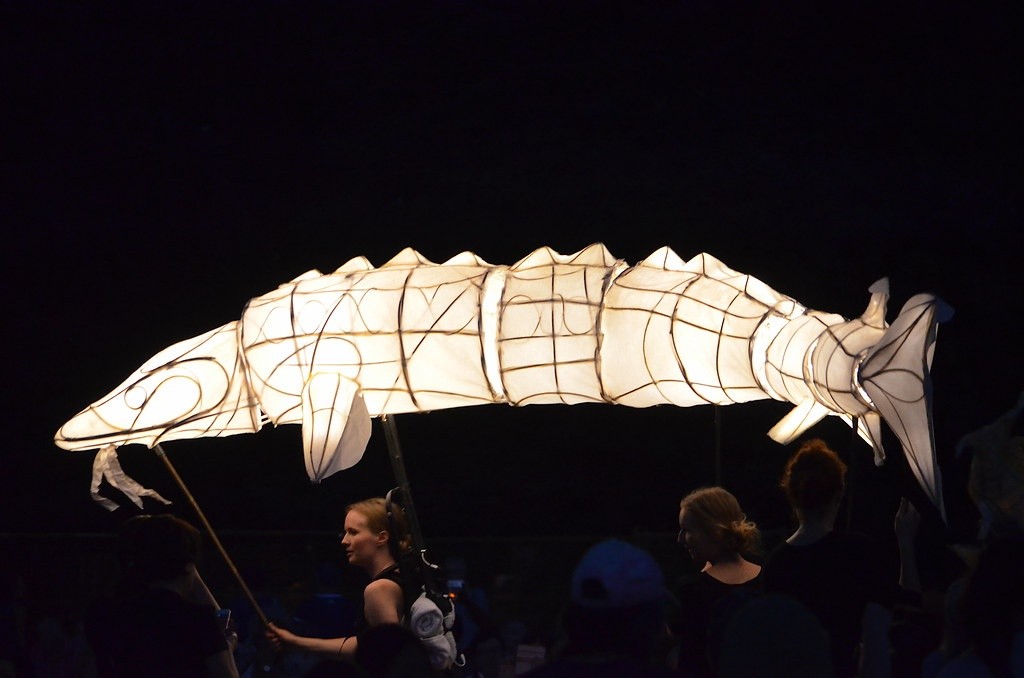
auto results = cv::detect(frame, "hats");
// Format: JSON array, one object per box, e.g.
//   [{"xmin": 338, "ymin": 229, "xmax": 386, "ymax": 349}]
[{"xmin": 572, "ymin": 539, "xmax": 667, "ymax": 610}]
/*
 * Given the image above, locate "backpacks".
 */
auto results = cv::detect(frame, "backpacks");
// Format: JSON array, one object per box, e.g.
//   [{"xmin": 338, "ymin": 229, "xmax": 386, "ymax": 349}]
[{"xmin": 401, "ymin": 587, "xmax": 467, "ymax": 672}]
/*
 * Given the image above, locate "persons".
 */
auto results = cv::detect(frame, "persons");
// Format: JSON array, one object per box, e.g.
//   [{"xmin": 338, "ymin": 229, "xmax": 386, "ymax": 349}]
[
  {"xmin": 84, "ymin": 513, "xmax": 241, "ymax": 678},
  {"xmin": 265, "ymin": 497, "xmax": 439, "ymax": 678},
  {"xmin": 663, "ymin": 486, "xmax": 770, "ymax": 678},
  {"xmin": 514, "ymin": 537, "xmax": 676, "ymax": 678},
  {"xmin": 765, "ymin": 440, "xmax": 921, "ymax": 678}
]
